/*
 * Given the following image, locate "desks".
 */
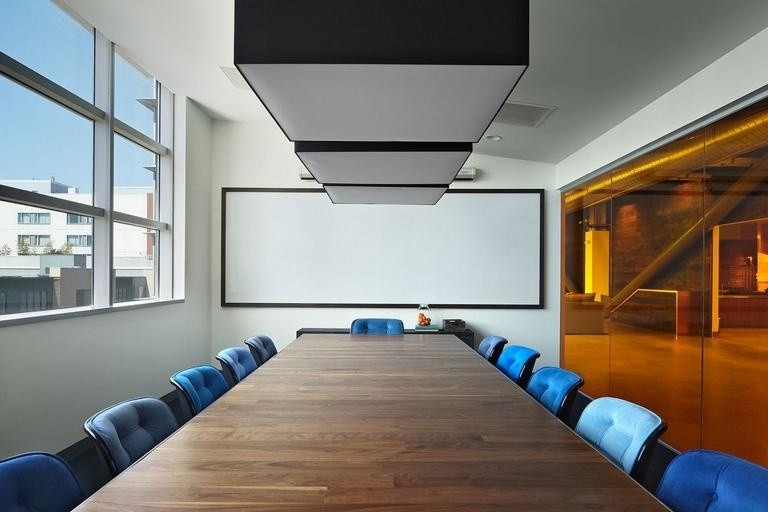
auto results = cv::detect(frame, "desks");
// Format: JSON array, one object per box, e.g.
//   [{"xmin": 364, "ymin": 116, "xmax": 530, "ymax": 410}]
[{"xmin": 66, "ymin": 334, "xmax": 674, "ymax": 511}]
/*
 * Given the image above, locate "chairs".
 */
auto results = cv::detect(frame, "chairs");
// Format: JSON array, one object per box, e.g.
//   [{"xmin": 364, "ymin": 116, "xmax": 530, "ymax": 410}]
[
  {"xmin": 497, "ymin": 345, "xmax": 541, "ymax": 389},
  {"xmin": 169, "ymin": 363, "xmax": 229, "ymax": 416},
  {"xmin": 524, "ymin": 367, "xmax": 584, "ymax": 421},
  {"xmin": 660, "ymin": 454, "xmax": 766, "ymax": 511},
  {"xmin": 564, "ymin": 301, "xmax": 603, "ymax": 336},
  {"xmin": 83, "ymin": 396, "xmax": 181, "ymax": 478},
  {"xmin": 479, "ymin": 336, "xmax": 508, "ymax": 365},
  {"xmin": 351, "ymin": 319, "xmax": 404, "ymax": 335},
  {"xmin": 245, "ymin": 334, "xmax": 279, "ymax": 367},
  {"xmin": 216, "ymin": 347, "xmax": 259, "ymax": 381},
  {"xmin": 576, "ymin": 396, "xmax": 666, "ymax": 476},
  {"xmin": 1, "ymin": 450, "xmax": 81, "ymax": 512}
]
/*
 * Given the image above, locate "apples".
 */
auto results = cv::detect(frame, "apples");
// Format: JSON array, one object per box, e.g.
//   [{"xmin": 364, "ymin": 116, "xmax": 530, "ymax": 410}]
[{"xmin": 419, "ymin": 313, "xmax": 430, "ymax": 326}]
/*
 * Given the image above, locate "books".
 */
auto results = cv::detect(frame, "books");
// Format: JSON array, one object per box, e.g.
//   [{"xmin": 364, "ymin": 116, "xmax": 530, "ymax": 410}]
[
  {"xmin": 415, "ymin": 324, "xmax": 440, "ymax": 329},
  {"xmin": 415, "ymin": 330, "xmax": 439, "ymax": 332}
]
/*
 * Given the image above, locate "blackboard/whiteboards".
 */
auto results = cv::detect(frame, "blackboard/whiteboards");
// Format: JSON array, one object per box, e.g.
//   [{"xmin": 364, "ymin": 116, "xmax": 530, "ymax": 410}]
[{"xmin": 221, "ymin": 187, "xmax": 544, "ymax": 308}]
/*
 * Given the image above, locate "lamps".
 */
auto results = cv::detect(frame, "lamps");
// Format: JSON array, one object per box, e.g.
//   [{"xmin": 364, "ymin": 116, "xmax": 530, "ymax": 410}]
[
  {"xmin": 321, "ymin": 184, "xmax": 450, "ymax": 204},
  {"xmin": 294, "ymin": 141, "xmax": 474, "ymax": 188},
  {"xmin": 232, "ymin": 1, "xmax": 532, "ymax": 144}
]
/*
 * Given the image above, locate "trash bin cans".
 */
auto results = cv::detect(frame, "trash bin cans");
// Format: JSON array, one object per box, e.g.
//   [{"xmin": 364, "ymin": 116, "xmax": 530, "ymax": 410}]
[{"xmin": 678, "ymin": 291, "xmax": 702, "ymax": 337}]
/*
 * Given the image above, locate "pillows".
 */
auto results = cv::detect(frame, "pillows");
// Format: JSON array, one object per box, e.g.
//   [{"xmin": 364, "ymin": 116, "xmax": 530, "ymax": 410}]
[{"xmin": 569, "ymin": 292, "xmax": 596, "ymax": 303}]
